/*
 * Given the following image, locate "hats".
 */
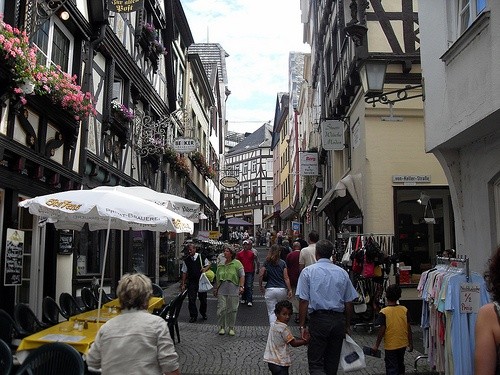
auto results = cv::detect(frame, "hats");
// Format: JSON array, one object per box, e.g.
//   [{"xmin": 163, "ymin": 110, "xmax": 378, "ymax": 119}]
[{"xmin": 243, "ymin": 241, "xmax": 249, "ymax": 245}]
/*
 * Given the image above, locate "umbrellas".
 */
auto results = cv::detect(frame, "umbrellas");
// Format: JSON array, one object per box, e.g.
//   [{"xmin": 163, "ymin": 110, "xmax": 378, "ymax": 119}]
[
  {"xmin": 220, "ymin": 217, "xmax": 253, "ymax": 227},
  {"xmin": 31, "ymin": 183, "xmax": 203, "ymax": 229},
  {"xmin": 20, "ymin": 187, "xmax": 193, "ymax": 321}
]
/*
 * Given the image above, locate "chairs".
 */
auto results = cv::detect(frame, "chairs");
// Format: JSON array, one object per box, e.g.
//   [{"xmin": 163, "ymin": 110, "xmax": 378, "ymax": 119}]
[{"xmin": 0, "ymin": 283, "xmax": 188, "ymax": 375}]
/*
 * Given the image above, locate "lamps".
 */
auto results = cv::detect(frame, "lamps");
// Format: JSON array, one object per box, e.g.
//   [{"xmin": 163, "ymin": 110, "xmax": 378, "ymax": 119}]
[
  {"xmin": 355, "ymin": 58, "xmax": 425, "ymax": 107},
  {"xmin": 416, "ymin": 192, "xmax": 430, "ymax": 206}
]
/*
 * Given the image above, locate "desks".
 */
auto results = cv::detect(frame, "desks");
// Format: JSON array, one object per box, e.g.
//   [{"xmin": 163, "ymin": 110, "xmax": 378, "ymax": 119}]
[
  {"xmin": 15, "ymin": 321, "xmax": 105, "ymax": 375},
  {"xmin": 102, "ymin": 296, "xmax": 164, "ymax": 313},
  {"xmin": 69, "ymin": 308, "xmax": 121, "ymax": 323}
]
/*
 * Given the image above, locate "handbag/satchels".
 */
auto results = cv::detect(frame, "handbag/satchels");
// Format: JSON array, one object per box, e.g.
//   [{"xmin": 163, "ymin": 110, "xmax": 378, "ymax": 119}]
[
  {"xmin": 342, "ymin": 235, "xmax": 387, "ymax": 319},
  {"xmin": 340, "ymin": 334, "xmax": 366, "ymax": 372},
  {"xmin": 262, "ymin": 260, "xmax": 268, "ymax": 282},
  {"xmin": 198, "ymin": 273, "xmax": 213, "ymax": 292},
  {"xmin": 424, "ymin": 200, "xmax": 436, "ymax": 225},
  {"xmin": 199, "ymin": 254, "xmax": 216, "ymax": 283}
]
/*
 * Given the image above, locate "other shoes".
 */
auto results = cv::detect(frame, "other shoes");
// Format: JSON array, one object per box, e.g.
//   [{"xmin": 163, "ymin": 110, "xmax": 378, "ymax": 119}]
[
  {"xmin": 189, "ymin": 316, "xmax": 197, "ymax": 323},
  {"xmin": 219, "ymin": 328, "xmax": 226, "ymax": 335},
  {"xmin": 200, "ymin": 309, "xmax": 208, "ymax": 319},
  {"xmin": 248, "ymin": 302, "xmax": 253, "ymax": 307},
  {"xmin": 306, "ymin": 316, "xmax": 311, "ymax": 322},
  {"xmin": 241, "ymin": 300, "xmax": 246, "ymax": 304},
  {"xmin": 294, "ymin": 316, "xmax": 299, "ymax": 323},
  {"xmin": 228, "ymin": 330, "xmax": 234, "ymax": 336}
]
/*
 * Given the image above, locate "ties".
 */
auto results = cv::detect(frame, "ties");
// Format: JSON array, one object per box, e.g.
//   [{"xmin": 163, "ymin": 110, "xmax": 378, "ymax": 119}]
[{"xmin": 193, "ymin": 257, "xmax": 194, "ymax": 262}]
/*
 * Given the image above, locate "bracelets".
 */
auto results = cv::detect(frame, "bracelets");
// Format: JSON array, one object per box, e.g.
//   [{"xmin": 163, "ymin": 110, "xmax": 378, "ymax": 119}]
[{"xmin": 298, "ymin": 326, "xmax": 307, "ymax": 330}]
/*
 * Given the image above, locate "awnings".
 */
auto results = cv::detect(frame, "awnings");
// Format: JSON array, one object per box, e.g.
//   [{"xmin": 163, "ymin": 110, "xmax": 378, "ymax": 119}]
[
  {"xmin": 316, "ymin": 174, "xmax": 363, "ymax": 225},
  {"xmin": 263, "ymin": 212, "xmax": 280, "ymax": 222}
]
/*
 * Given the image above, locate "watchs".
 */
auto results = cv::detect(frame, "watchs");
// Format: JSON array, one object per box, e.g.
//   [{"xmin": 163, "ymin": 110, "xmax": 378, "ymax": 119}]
[{"xmin": 240, "ymin": 285, "xmax": 244, "ymax": 289}]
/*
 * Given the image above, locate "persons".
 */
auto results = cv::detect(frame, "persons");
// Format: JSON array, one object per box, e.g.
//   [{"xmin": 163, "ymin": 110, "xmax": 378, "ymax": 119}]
[
  {"xmin": 285, "ymin": 241, "xmax": 306, "ymax": 323},
  {"xmin": 472, "ymin": 245, "xmax": 500, "ymax": 375},
  {"xmin": 262, "ymin": 300, "xmax": 308, "ymax": 375},
  {"xmin": 86, "ymin": 273, "xmax": 180, "ymax": 375},
  {"xmin": 213, "ymin": 246, "xmax": 245, "ymax": 335},
  {"xmin": 237, "ymin": 239, "xmax": 260, "ymax": 307},
  {"xmin": 298, "ymin": 231, "xmax": 333, "ymax": 273},
  {"xmin": 259, "ymin": 245, "xmax": 292, "ymax": 328},
  {"xmin": 230, "ymin": 228, "xmax": 255, "ymax": 248},
  {"xmin": 296, "ymin": 239, "xmax": 361, "ymax": 375},
  {"xmin": 181, "ymin": 244, "xmax": 210, "ymax": 322},
  {"xmin": 256, "ymin": 226, "xmax": 308, "ymax": 261},
  {"xmin": 374, "ymin": 284, "xmax": 413, "ymax": 375}
]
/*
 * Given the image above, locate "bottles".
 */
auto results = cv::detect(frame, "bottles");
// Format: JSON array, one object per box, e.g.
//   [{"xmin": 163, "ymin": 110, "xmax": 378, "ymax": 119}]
[
  {"xmin": 79, "ymin": 322, "xmax": 83, "ymax": 331},
  {"xmin": 84, "ymin": 320, "xmax": 88, "ymax": 329},
  {"xmin": 108, "ymin": 305, "xmax": 111, "ymax": 313},
  {"xmin": 73, "ymin": 319, "xmax": 78, "ymax": 329},
  {"xmin": 111, "ymin": 305, "xmax": 117, "ymax": 314}
]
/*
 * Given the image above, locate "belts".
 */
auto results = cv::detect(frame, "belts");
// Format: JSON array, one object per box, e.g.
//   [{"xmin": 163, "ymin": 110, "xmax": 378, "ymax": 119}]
[{"xmin": 309, "ymin": 310, "xmax": 343, "ymax": 315}]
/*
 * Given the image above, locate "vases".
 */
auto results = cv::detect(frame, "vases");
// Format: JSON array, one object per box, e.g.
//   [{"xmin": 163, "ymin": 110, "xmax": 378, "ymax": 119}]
[{"xmin": 111, "ymin": 111, "xmax": 129, "ymax": 130}]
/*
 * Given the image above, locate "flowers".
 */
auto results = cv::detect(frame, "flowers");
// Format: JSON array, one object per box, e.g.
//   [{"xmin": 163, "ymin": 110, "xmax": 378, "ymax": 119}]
[
  {"xmin": 143, "ymin": 23, "xmax": 169, "ymax": 56},
  {"xmin": 111, "ymin": 99, "xmax": 134, "ymax": 122},
  {"xmin": 0, "ymin": 13, "xmax": 99, "ymax": 121},
  {"xmin": 194, "ymin": 151, "xmax": 216, "ymax": 178},
  {"xmin": 176, "ymin": 155, "xmax": 191, "ymax": 181}
]
